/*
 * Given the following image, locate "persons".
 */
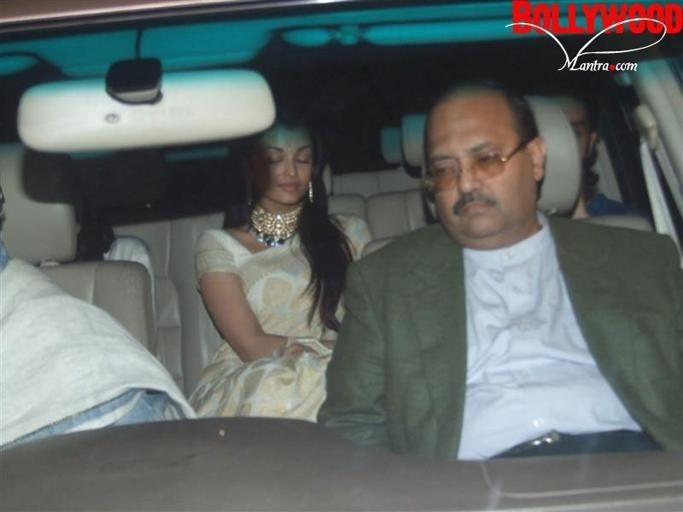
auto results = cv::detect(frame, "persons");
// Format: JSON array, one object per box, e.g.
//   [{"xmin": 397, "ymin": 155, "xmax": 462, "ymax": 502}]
[
  {"xmin": 317, "ymin": 75, "xmax": 683, "ymax": 461},
  {"xmin": 39, "ymin": 184, "xmax": 157, "ymax": 333},
  {"xmin": 187, "ymin": 97, "xmax": 375, "ymax": 424},
  {"xmin": 542, "ymin": 88, "xmax": 655, "ymax": 231}
]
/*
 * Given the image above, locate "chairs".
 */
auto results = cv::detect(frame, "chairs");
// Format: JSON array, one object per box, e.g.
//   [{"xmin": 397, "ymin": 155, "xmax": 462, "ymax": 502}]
[{"xmin": 1, "ymin": 91, "xmax": 654, "ymax": 399}]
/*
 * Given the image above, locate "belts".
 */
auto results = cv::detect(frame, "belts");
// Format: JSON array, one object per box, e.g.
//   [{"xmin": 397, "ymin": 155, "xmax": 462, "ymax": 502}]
[{"xmin": 495, "ymin": 430, "xmax": 661, "ymax": 455}]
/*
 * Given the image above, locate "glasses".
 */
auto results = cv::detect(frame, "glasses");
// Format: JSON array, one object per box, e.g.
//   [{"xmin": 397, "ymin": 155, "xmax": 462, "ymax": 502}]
[{"xmin": 424, "ymin": 142, "xmax": 525, "ymax": 193}]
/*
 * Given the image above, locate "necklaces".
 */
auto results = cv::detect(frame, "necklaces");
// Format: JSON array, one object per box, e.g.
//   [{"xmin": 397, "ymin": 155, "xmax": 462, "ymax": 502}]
[{"xmin": 246, "ymin": 202, "xmax": 304, "ymax": 247}]
